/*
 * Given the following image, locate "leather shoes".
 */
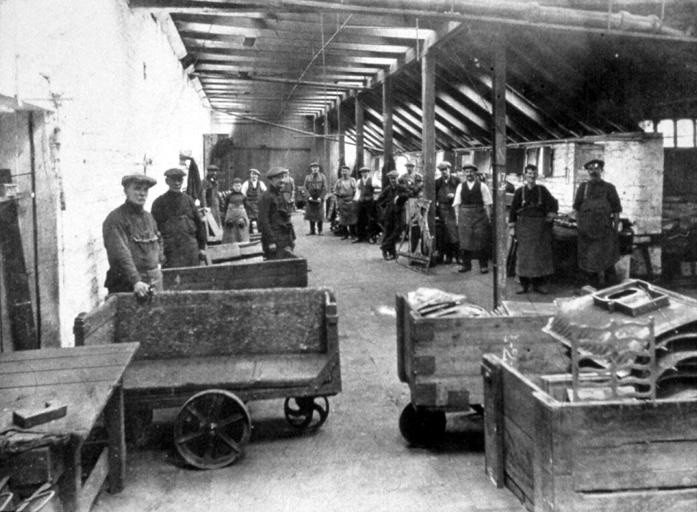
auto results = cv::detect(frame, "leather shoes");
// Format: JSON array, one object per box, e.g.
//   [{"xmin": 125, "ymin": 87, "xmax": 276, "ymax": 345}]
[
  {"xmin": 305, "ymin": 230, "xmax": 316, "ymax": 236},
  {"xmin": 458, "ymin": 265, "xmax": 472, "ymax": 273},
  {"xmin": 533, "ymin": 286, "xmax": 550, "ymax": 295},
  {"xmin": 339, "ymin": 235, "xmax": 348, "ymax": 240},
  {"xmin": 385, "ymin": 254, "xmax": 395, "ymax": 260},
  {"xmin": 382, "ymin": 250, "xmax": 388, "ymax": 258},
  {"xmin": 515, "ymin": 287, "xmax": 532, "ymax": 294},
  {"xmin": 480, "ymin": 265, "xmax": 490, "ymax": 274},
  {"xmin": 368, "ymin": 237, "xmax": 375, "ymax": 244},
  {"xmin": 351, "ymin": 236, "xmax": 363, "ymax": 243},
  {"xmin": 318, "ymin": 232, "xmax": 324, "ymax": 236}
]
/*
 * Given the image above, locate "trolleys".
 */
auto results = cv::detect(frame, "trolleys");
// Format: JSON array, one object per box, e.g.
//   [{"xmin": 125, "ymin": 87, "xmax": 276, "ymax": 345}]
[
  {"xmin": 74, "ymin": 287, "xmax": 343, "ymax": 473},
  {"xmin": 392, "ymin": 284, "xmax": 696, "ymax": 451}
]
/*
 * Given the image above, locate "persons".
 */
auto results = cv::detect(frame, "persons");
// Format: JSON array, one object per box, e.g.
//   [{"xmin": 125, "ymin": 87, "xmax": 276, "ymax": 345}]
[
  {"xmin": 333, "ymin": 163, "xmax": 424, "ymax": 260},
  {"xmin": 102, "ymin": 172, "xmax": 163, "ymax": 298},
  {"xmin": 304, "ymin": 163, "xmax": 327, "ymax": 236},
  {"xmin": 509, "ymin": 164, "xmax": 558, "ymax": 295},
  {"xmin": 152, "ymin": 168, "xmax": 208, "ymax": 268},
  {"xmin": 574, "ymin": 159, "xmax": 622, "ymax": 295},
  {"xmin": 436, "ymin": 163, "xmax": 493, "ymax": 272},
  {"xmin": 204, "ymin": 164, "xmax": 266, "ymax": 243},
  {"xmin": 258, "ymin": 166, "xmax": 297, "ymax": 259}
]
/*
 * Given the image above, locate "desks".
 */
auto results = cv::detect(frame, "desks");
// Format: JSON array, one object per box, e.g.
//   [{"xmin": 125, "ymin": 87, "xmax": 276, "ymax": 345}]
[{"xmin": 0, "ymin": 340, "xmax": 141, "ymax": 512}]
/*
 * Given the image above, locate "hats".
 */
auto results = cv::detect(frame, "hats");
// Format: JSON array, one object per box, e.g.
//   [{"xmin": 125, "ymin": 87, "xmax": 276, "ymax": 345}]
[
  {"xmin": 163, "ymin": 168, "xmax": 187, "ymax": 177},
  {"xmin": 230, "ymin": 177, "xmax": 243, "ymax": 184},
  {"xmin": 248, "ymin": 168, "xmax": 261, "ymax": 176},
  {"xmin": 308, "ymin": 161, "xmax": 320, "ymax": 168},
  {"xmin": 436, "ymin": 161, "xmax": 453, "ymax": 170},
  {"xmin": 358, "ymin": 166, "xmax": 371, "ymax": 172},
  {"xmin": 385, "ymin": 169, "xmax": 400, "ymax": 177},
  {"xmin": 462, "ymin": 163, "xmax": 479, "ymax": 171},
  {"xmin": 340, "ymin": 165, "xmax": 350, "ymax": 170},
  {"xmin": 264, "ymin": 166, "xmax": 290, "ymax": 178},
  {"xmin": 206, "ymin": 165, "xmax": 220, "ymax": 172},
  {"xmin": 121, "ymin": 173, "xmax": 158, "ymax": 187},
  {"xmin": 583, "ymin": 159, "xmax": 605, "ymax": 170},
  {"xmin": 404, "ymin": 162, "xmax": 416, "ymax": 167}
]
